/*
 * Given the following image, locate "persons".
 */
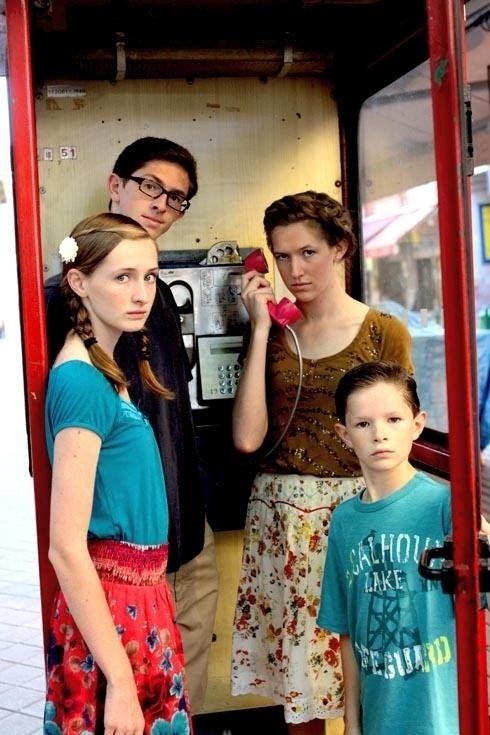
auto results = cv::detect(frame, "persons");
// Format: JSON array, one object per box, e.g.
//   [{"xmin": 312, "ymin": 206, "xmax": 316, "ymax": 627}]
[
  {"xmin": 315, "ymin": 357, "xmax": 462, "ymax": 735},
  {"xmin": 43, "ymin": 136, "xmax": 223, "ymax": 721},
  {"xmin": 39, "ymin": 211, "xmax": 197, "ymax": 735},
  {"xmin": 228, "ymin": 187, "xmax": 413, "ymax": 734}
]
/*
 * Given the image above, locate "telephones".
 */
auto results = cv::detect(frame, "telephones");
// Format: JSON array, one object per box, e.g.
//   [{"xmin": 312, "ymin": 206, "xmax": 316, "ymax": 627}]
[{"xmin": 244, "ymin": 248, "xmax": 303, "ymax": 329}]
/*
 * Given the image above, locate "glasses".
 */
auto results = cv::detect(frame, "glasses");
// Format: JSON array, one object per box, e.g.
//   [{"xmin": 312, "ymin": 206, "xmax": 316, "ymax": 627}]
[{"xmin": 119, "ymin": 174, "xmax": 190, "ymax": 214}]
